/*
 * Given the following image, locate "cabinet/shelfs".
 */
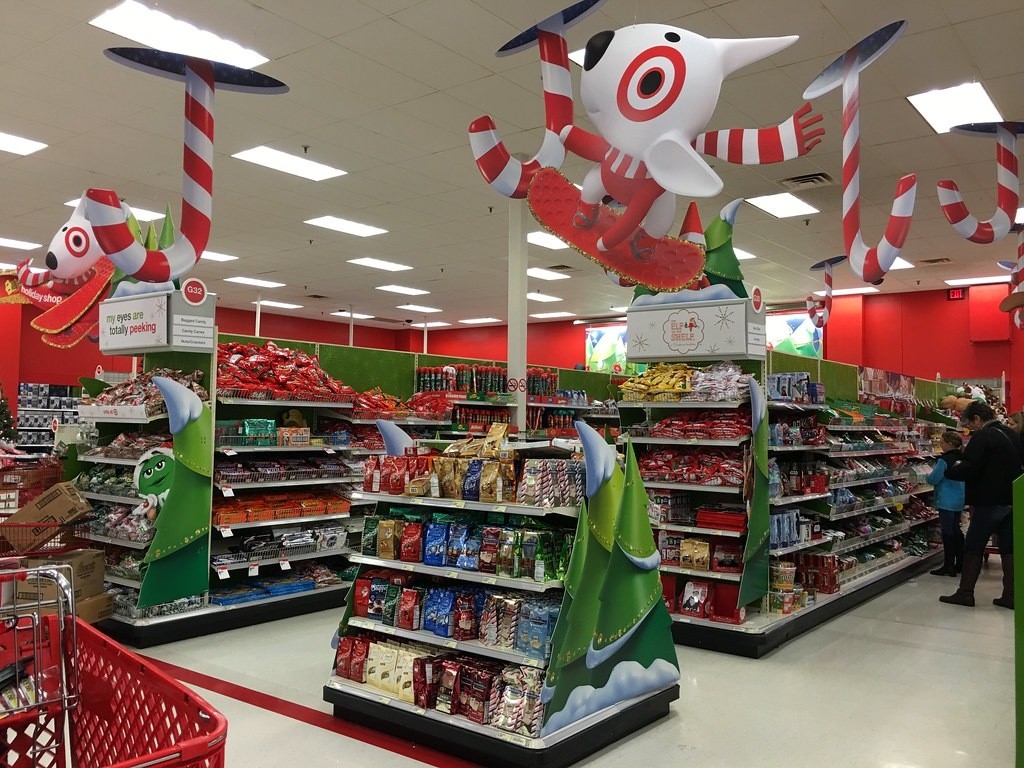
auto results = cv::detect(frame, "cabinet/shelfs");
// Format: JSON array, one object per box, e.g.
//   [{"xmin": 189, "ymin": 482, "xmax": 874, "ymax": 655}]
[{"xmin": 0, "ymin": 278, "xmax": 1024, "ymax": 768}]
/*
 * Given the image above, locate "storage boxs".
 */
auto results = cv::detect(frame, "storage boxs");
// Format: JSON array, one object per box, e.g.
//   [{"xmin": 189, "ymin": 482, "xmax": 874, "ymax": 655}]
[
  {"xmin": 14, "ymin": 548, "xmax": 106, "ymax": 603},
  {"xmin": 0, "ymin": 481, "xmax": 92, "ymax": 556},
  {"xmin": 3, "ymin": 593, "xmax": 114, "ymax": 634}
]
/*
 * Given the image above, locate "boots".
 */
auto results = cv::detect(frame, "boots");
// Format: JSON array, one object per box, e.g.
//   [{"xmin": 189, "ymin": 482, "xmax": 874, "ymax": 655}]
[
  {"xmin": 955, "ymin": 534, "xmax": 964, "ymax": 573},
  {"xmin": 993, "ymin": 554, "xmax": 1014, "ymax": 609},
  {"xmin": 939, "ymin": 553, "xmax": 982, "ymax": 606},
  {"xmin": 930, "ymin": 535, "xmax": 956, "ymax": 577}
]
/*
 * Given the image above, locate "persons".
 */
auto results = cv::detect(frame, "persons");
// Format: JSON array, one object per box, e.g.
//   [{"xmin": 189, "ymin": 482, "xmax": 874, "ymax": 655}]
[{"xmin": 925, "ymin": 400, "xmax": 1024, "ymax": 611}]
